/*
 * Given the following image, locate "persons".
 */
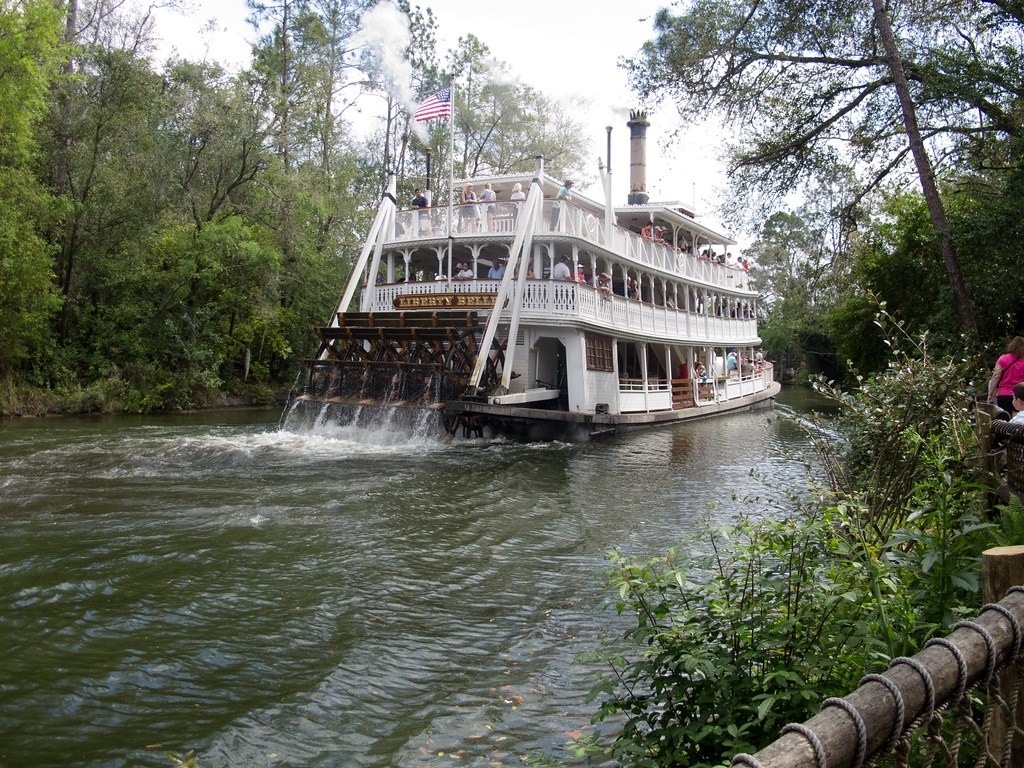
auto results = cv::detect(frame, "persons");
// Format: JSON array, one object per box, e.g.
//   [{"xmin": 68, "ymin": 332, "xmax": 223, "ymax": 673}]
[
  {"xmin": 717, "ymin": 298, "xmax": 755, "ymax": 318},
  {"xmin": 661, "ymin": 295, "xmax": 675, "ymax": 309},
  {"xmin": 462, "ymin": 183, "xmax": 477, "ymax": 232},
  {"xmin": 677, "ymin": 359, "xmax": 707, "ymax": 395},
  {"xmin": 529, "ymin": 264, "xmax": 535, "ymax": 278},
  {"xmin": 510, "ymin": 183, "xmax": 525, "ymax": 226},
  {"xmin": 727, "ymin": 349, "xmax": 766, "ymax": 381},
  {"xmin": 409, "ymin": 274, "xmax": 417, "ymax": 281},
  {"xmin": 488, "ymin": 258, "xmax": 505, "ymax": 278},
  {"xmin": 395, "ymin": 216, "xmax": 405, "ymax": 239},
  {"xmin": 480, "ymin": 183, "xmax": 496, "ymax": 233},
  {"xmin": 988, "ymin": 336, "xmax": 1024, "ymax": 497},
  {"xmin": 365, "ymin": 273, "xmax": 369, "ymax": 283},
  {"xmin": 376, "ymin": 273, "xmax": 384, "ymax": 285},
  {"xmin": 453, "ymin": 263, "xmax": 474, "ymax": 280},
  {"xmin": 640, "ymin": 221, "xmax": 750, "ymax": 271},
  {"xmin": 621, "ymin": 277, "xmax": 639, "ymax": 301},
  {"xmin": 550, "ymin": 180, "xmax": 574, "ymax": 232},
  {"xmin": 546, "ymin": 254, "xmax": 612, "ymax": 300},
  {"xmin": 412, "ymin": 189, "xmax": 432, "ymax": 236}
]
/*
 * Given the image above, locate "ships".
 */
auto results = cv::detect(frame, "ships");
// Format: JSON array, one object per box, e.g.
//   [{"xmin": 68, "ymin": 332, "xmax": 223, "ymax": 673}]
[{"xmin": 293, "ymin": 66, "xmax": 783, "ymax": 446}]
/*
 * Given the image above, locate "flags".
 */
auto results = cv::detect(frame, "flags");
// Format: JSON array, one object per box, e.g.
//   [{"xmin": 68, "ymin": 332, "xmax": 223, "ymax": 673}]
[{"xmin": 414, "ymin": 88, "xmax": 450, "ymax": 121}]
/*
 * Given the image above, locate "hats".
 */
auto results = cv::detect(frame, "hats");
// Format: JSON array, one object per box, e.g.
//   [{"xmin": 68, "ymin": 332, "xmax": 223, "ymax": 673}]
[
  {"xmin": 560, "ymin": 255, "xmax": 571, "ymax": 262},
  {"xmin": 577, "ymin": 264, "xmax": 585, "ymax": 268}
]
